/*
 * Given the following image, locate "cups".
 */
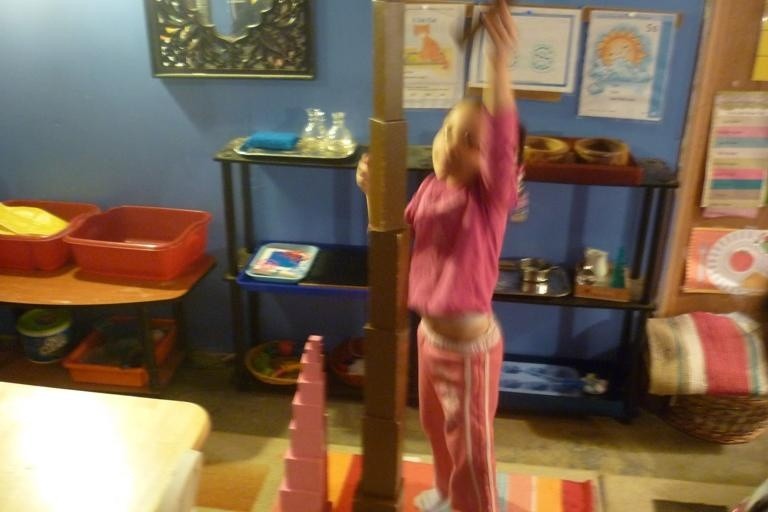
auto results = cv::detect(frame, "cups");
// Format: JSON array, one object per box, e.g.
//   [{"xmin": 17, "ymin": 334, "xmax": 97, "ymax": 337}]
[{"xmin": 583, "ymin": 247, "xmax": 609, "ymax": 277}]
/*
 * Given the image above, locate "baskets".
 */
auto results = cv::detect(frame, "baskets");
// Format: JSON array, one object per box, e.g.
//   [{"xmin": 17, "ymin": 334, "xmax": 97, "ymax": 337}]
[{"xmin": 657, "ymin": 389, "xmax": 768, "ymax": 445}]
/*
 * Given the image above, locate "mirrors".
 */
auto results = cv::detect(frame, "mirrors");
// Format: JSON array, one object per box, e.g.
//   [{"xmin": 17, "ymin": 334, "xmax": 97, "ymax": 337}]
[{"xmin": 144, "ymin": 0, "xmax": 316, "ymax": 80}]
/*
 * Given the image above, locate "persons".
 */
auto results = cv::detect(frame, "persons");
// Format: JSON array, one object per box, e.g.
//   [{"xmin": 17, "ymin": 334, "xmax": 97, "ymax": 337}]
[{"xmin": 357, "ymin": 0, "xmax": 527, "ymax": 512}]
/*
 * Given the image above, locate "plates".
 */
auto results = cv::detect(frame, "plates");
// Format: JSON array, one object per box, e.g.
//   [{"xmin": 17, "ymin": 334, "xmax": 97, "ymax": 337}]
[{"xmin": 245, "ymin": 339, "xmax": 300, "ymax": 385}]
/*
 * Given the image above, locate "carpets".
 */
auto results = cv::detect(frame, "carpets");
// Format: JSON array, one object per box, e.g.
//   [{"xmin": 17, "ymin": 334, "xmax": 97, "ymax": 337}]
[{"xmin": 327, "ymin": 450, "xmax": 605, "ymax": 511}]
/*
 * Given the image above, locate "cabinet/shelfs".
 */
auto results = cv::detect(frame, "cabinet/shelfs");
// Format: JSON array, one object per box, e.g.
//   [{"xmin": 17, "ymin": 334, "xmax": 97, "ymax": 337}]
[{"xmin": 213, "ymin": 138, "xmax": 682, "ymax": 424}]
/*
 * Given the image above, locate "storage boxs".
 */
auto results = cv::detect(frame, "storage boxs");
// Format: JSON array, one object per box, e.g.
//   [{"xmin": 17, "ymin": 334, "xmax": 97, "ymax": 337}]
[
  {"xmin": 61, "ymin": 205, "xmax": 212, "ymax": 280},
  {"xmin": 0, "ymin": 199, "xmax": 102, "ymax": 272}
]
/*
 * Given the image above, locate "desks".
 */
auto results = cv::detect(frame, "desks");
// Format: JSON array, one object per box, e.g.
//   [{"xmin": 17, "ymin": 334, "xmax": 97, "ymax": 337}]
[
  {"xmin": 0, "ymin": 255, "xmax": 217, "ymax": 393},
  {"xmin": 1, "ymin": 382, "xmax": 212, "ymax": 512}
]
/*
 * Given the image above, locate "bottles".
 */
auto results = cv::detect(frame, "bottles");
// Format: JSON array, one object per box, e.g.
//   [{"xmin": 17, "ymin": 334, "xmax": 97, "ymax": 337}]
[
  {"xmin": 611, "ymin": 248, "xmax": 628, "ymax": 288},
  {"xmin": 299, "ymin": 107, "xmax": 328, "ymax": 155},
  {"xmin": 328, "ymin": 112, "xmax": 354, "ymax": 155}
]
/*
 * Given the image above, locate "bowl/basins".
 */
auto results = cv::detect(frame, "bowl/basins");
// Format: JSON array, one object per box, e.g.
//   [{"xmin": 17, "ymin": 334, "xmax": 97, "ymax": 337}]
[
  {"xmin": 573, "ymin": 138, "xmax": 629, "ymax": 167},
  {"xmin": 329, "ymin": 346, "xmax": 366, "ymax": 389},
  {"xmin": 522, "ymin": 136, "xmax": 569, "ymax": 163},
  {"xmin": 520, "ymin": 256, "xmax": 559, "ymax": 282}
]
